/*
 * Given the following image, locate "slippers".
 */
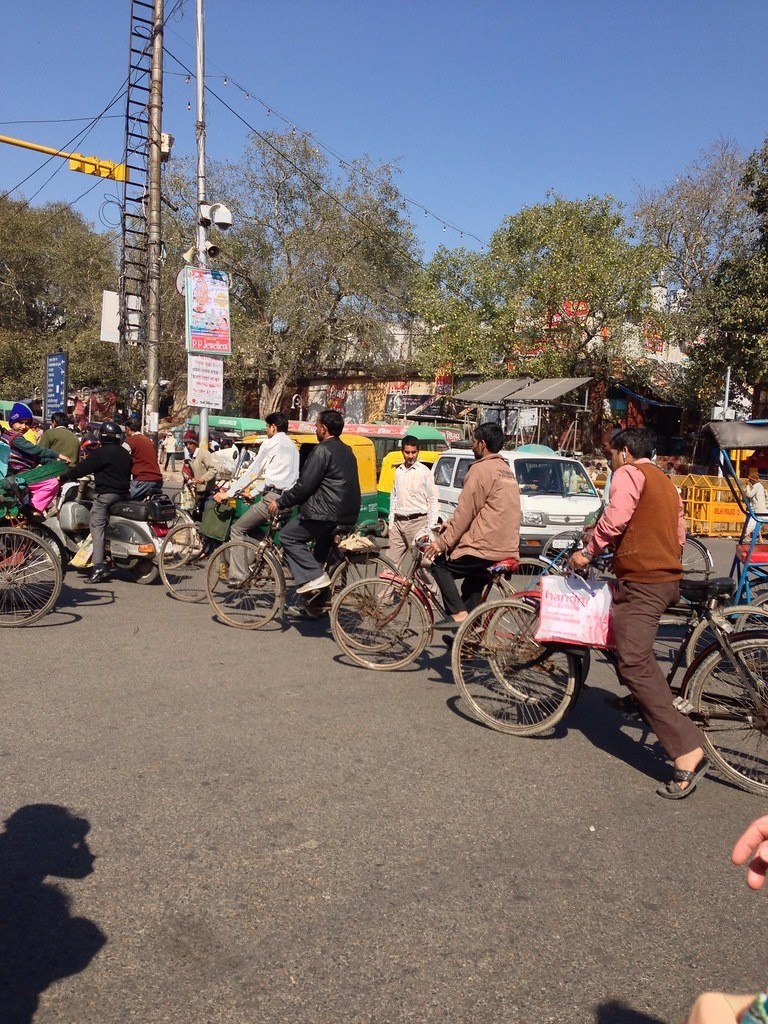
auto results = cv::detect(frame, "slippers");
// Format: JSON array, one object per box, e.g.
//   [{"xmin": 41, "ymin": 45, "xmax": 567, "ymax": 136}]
[
  {"xmin": 657, "ymin": 758, "xmax": 711, "ymax": 800},
  {"xmin": 607, "ymin": 691, "xmax": 642, "ymax": 711},
  {"xmin": 442, "ymin": 633, "xmax": 475, "ymax": 655},
  {"xmin": 430, "ymin": 615, "xmax": 464, "ymax": 628}
]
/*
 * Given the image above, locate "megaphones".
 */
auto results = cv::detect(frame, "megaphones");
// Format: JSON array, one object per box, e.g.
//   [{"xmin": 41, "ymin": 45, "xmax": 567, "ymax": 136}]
[
  {"xmin": 182, "ymin": 247, "xmax": 196, "ymax": 264},
  {"xmin": 204, "ymin": 240, "xmax": 220, "ymax": 259}
]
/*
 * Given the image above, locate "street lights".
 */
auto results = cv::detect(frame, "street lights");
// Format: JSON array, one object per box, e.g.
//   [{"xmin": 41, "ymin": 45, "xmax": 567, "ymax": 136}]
[
  {"xmin": 79, "ymin": 386, "xmax": 92, "ymax": 423},
  {"xmin": 132, "ymin": 390, "xmax": 145, "ymax": 435},
  {"xmin": 181, "ymin": 203, "xmax": 234, "ymax": 451},
  {"xmin": 291, "ymin": 394, "xmax": 303, "ymax": 422},
  {"xmin": 392, "ymin": 393, "xmax": 407, "ymax": 422}
]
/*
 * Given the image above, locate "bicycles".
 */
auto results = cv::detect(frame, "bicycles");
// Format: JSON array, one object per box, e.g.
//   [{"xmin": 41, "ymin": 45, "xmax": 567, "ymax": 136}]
[
  {"xmin": 1, "ymin": 399, "xmax": 767, "ymax": 723},
  {"xmin": 452, "ymin": 545, "xmax": 768, "ymax": 800}
]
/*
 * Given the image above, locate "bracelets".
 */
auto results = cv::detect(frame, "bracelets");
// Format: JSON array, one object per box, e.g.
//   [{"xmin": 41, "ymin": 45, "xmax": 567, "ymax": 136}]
[{"xmin": 582, "ymin": 548, "xmax": 592, "ymax": 560}]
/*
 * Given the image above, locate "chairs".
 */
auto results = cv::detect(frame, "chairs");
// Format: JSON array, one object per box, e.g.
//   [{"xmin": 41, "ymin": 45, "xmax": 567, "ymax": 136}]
[{"xmin": 528, "ymin": 467, "xmax": 552, "ymax": 489}]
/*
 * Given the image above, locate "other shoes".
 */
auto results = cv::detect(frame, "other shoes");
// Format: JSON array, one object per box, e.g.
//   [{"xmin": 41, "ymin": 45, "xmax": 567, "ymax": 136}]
[
  {"xmin": 424, "ymin": 596, "xmax": 440, "ymax": 610},
  {"xmin": 743, "ymin": 536, "xmax": 752, "ymax": 542},
  {"xmin": 376, "ymin": 589, "xmax": 401, "ymax": 606},
  {"xmin": 163, "ymin": 468, "xmax": 166, "ymax": 471},
  {"xmin": 757, "ymin": 539, "xmax": 765, "ymax": 543},
  {"xmin": 173, "ymin": 470, "xmax": 179, "ymax": 472},
  {"xmin": 220, "ymin": 575, "xmax": 244, "ymax": 587}
]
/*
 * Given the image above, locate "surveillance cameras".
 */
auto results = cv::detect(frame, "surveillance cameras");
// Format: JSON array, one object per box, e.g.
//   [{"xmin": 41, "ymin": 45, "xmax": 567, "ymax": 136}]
[{"xmin": 215, "ymin": 209, "xmax": 233, "ymax": 230}]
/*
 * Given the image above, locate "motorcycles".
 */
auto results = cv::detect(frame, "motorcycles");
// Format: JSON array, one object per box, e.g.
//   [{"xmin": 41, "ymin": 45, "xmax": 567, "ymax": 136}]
[
  {"xmin": 40, "ymin": 473, "xmax": 178, "ymax": 584},
  {"xmin": 367, "ymin": 449, "xmax": 443, "ymax": 539},
  {"xmin": 214, "ymin": 434, "xmax": 382, "ymax": 564}
]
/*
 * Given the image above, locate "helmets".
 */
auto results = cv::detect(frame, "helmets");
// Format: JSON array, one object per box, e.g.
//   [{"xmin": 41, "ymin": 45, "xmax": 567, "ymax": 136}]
[
  {"xmin": 98, "ymin": 422, "xmax": 123, "ymax": 445},
  {"xmin": 81, "ymin": 433, "xmax": 99, "ymax": 449}
]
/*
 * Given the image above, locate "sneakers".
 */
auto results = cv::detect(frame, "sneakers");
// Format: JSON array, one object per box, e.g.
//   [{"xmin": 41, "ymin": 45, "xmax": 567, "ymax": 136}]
[
  {"xmin": 83, "ymin": 562, "xmax": 113, "ymax": 584},
  {"xmin": 289, "ymin": 603, "xmax": 326, "ymax": 618},
  {"xmin": 296, "ymin": 571, "xmax": 331, "ymax": 594}
]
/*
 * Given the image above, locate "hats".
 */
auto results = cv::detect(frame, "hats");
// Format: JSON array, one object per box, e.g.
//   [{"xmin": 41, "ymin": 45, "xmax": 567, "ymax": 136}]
[
  {"xmin": 8, "ymin": 402, "xmax": 33, "ymax": 427},
  {"xmin": 183, "ymin": 430, "xmax": 199, "ymax": 447},
  {"xmin": 168, "ymin": 433, "xmax": 172, "ymax": 435},
  {"xmin": 748, "ymin": 472, "xmax": 760, "ymax": 483}
]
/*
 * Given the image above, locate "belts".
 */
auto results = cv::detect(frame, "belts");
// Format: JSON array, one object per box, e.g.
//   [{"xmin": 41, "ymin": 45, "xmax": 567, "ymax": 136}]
[
  {"xmin": 397, "ymin": 513, "xmax": 428, "ymax": 521},
  {"xmin": 264, "ymin": 486, "xmax": 285, "ymax": 495}
]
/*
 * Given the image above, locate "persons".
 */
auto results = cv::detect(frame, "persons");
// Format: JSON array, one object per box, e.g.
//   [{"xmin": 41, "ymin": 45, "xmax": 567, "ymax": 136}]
[
  {"xmin": 375, "ymin": 436, "xmax": 442, "ymax": 610},
  {"xmin": 582, "ymin": 428, "xmax": 621, "ymax": 543},
  {"xmin": 518, "ymin": 471, "xmax": 537, "ymax": 490},
  {"xmin": 422, "ymin": 424, "xmax": 520, "ymax": 650},
  {"xmin": 213, "ymin": 412, "xmax": 300, "ymax": 585},
  {"xmin": 159, "ymin": 430, "xmax": 239, "ymax": 556},
  {"xmin": 121, "ymin": 417, "xmax": 163, "ymax": 500},
  {"xmin": 689, "ymin": 815, "xmax": 768, "ymax": 1024},
  {"xmin": 563, "ymin": 461, "xmax": 607, "ymax": 492},
  {"xmin": 665, "ymin": 462, "xmax": 676, "ymax": 474},
  {"xmin": 36, "ymin": 411, "xmax": 78, "ymax": 468},
  {"xmin": 0, "ymin": 402, "xmax": 71, "ymax": 520},
  {"xmin": 744, "ymin": 472, "xmax": 768, "ymax": 542},
  {"xmin": 268, "ymin": 410, "xmax": 359, "ymax": 593},
  {"xmin": 208, "ymin": 435, "xmax": 230, "ymax": 453},
  {"xmin": 158, "ymin": 436, "xmax": 164, "ymax": 464},
  {"xmin": 65, "ymin": 422, "xmax": 133, "ymax": 583},
  {"xmin": 566, "ymin": 429, "xmax": 711, "ymax": 798}
]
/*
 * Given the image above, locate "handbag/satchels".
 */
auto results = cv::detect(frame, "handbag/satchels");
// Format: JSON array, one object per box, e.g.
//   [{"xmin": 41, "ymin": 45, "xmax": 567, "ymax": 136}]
[
  {"xmin": 533, "ymin": 562, "xmax": 616, "ymax": 649},
  {"xmin": 199, "ymin": 500, "xmax": 235, "ymax": 542},
  {"xmin": 180, "ymin": 484, "xmax": 197, "ymax": 511}
]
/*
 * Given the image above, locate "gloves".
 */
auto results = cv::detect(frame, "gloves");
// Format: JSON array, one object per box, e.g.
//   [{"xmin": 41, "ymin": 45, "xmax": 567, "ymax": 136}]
[{"xmin": 60, "ymin": 474, "xmax": 69, "ymax": 482}]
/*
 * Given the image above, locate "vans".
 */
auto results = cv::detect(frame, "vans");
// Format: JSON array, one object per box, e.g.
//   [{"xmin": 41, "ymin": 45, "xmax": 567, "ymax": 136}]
[{"xmin": 431, "ymin": 448, "xmax": 603, "ymax": 575}]
